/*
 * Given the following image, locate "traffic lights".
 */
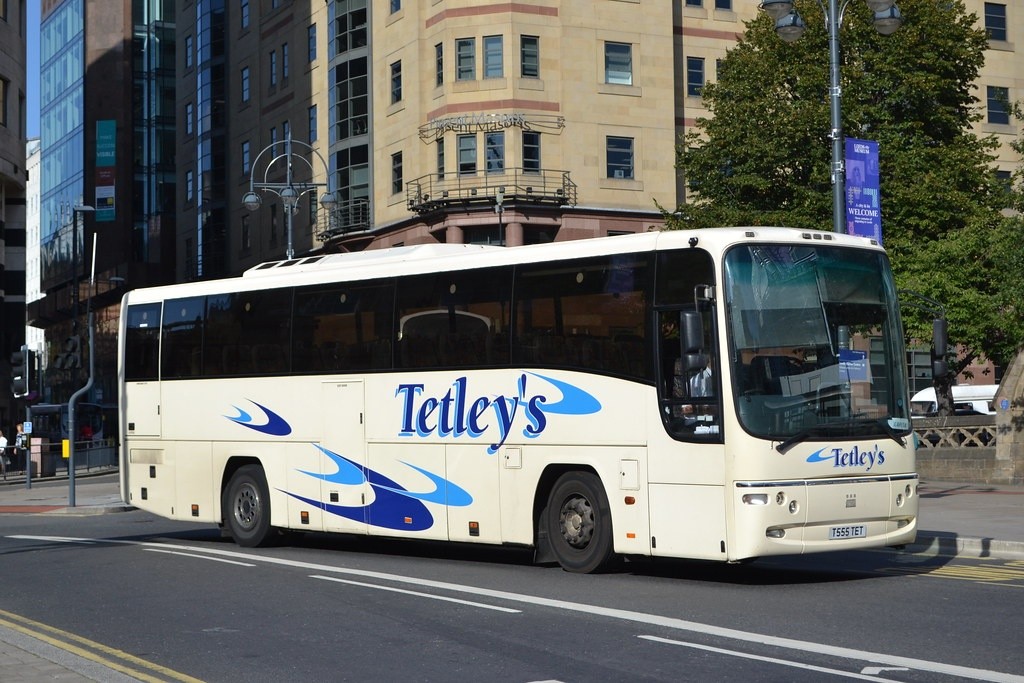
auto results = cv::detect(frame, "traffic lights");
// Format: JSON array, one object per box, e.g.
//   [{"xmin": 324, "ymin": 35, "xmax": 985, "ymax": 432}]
[
  {"xmin": 9, "ymin": 349, "xmax": 30, "ymax": 398},
  {"xmin": 63, "ymin": 335, "xmax": 82, "ymax": 369}
]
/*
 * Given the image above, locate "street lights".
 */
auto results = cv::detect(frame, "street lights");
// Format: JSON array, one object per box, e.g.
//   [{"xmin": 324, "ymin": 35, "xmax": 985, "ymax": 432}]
[
  {"xmin": 241, "ymin": 122, "xmax": 338, "ymax": 259},
  {"xmin": 70, "ymin": 204, "xmax": 95, "ymax": 392}
]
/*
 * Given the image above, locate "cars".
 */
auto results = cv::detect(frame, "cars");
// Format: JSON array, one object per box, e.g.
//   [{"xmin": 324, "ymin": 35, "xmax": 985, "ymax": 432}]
[{"xmin": 927, "ymin": 409, "xmax": 991, "ymax": 447}]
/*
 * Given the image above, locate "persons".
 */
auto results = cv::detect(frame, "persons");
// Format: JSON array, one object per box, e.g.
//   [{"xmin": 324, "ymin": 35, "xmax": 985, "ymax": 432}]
[
  {"xmin": 664, "ymin": 347, "xmax": 712, "ymax": 414},
  {"xmin": 80, "ymin": 425, "xmax": 93, "ymax": 448},
  {"xmin": 0, "ymin": 431, "xmax": 8, "ymax": 481},
  {"xmin": 14, "ymin": 424, "xmax": 26, "ymax": 475}
]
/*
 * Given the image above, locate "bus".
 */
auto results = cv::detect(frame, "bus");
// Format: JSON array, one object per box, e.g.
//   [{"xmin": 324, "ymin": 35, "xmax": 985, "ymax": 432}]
[
  {"xmin": 30, "ymin": 401, "xmax": 118, "ymax": 451},
  {"xmin": 115, "ymin": 224, "xmax": 951, "ymax": 574},
  {"xmin": 911, "ymin": 384, "xmax": 1000, "ymax": 417}
]
